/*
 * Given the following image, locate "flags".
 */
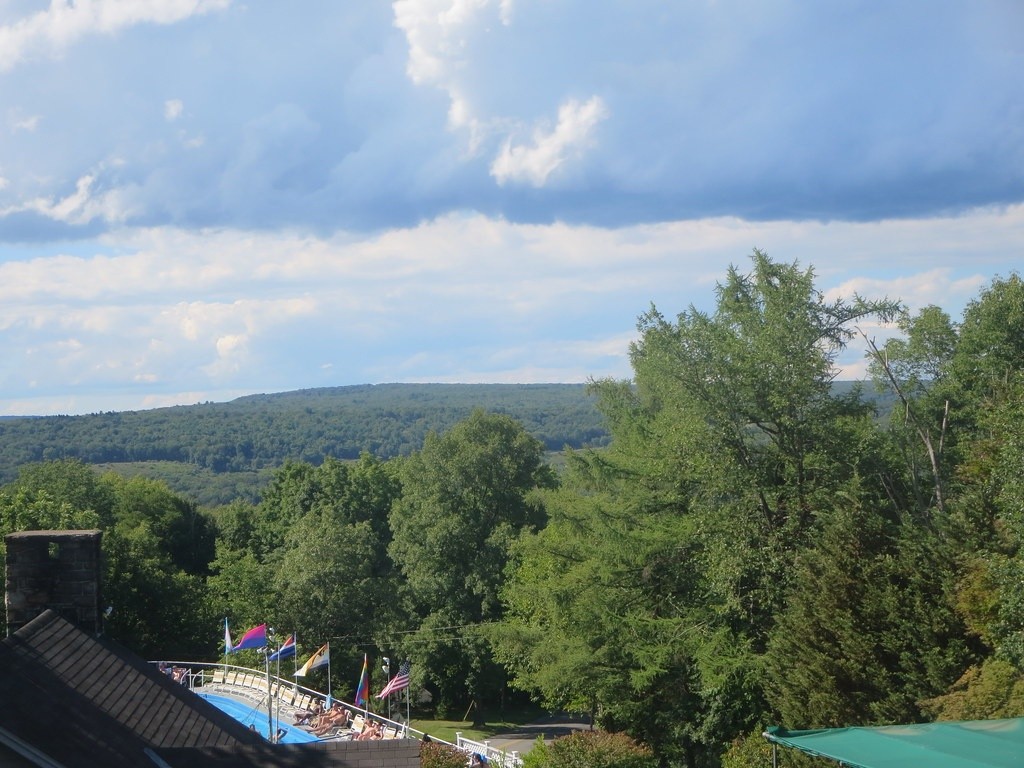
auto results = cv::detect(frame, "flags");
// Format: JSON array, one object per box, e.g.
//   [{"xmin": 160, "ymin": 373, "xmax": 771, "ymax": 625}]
[
  {"xmin": 355, "ymin": 664, "xmax": 368, "ymax": 705},
  {"xmin": 293, "ymin": 645, "xmax": 328, "ymax": 676},
  {"xmin": 232, "ymin": 625, "xmax": 266, "ymax": 650},
  {"xmin": 225, "ymin": 617, "xmax": 236, "ymax": 655},
  {"xmin": 268, "ymin": 636, "xmax": 295, "ymax": 660},
  {"xmin": 375, "ymin": 662, "xmax": 409, "ymax": 700}
]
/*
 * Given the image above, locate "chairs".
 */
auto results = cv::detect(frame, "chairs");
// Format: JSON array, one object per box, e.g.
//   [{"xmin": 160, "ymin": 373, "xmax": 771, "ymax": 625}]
[
  {"xmin": 159, "ymin": 667, "xmax": 186, "ymax": 686},
  {"xmin": 203, "ymin": 669, "xmax": 406, "ymax": 742}
]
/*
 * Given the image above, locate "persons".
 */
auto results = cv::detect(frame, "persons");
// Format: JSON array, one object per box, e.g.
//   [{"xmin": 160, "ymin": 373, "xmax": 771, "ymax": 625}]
[
  {"xmin": 159, "ymin": 662, "xmax": 181, "ymax": 683},
  {"xmin": 273, "ymin": 728, "xmax": 287, "ymax": 740},
  {"xmin": 469, "ymin": 752, "xmax": 489, "ymax": 768},
  {"xmin": 293, "ymin": 698, "xmax": 384, "ymax": 742},
  {"xmin": 250, "ymin": 724, "xmax": 255, "ymax": 730}
]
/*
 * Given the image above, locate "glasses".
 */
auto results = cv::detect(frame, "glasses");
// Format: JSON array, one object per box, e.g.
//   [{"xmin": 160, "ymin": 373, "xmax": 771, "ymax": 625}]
[{"xmin": 172, "ymin": 668, "xmax": 175, "ymax": 669}]
